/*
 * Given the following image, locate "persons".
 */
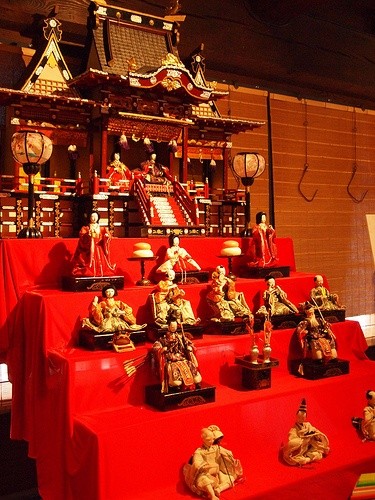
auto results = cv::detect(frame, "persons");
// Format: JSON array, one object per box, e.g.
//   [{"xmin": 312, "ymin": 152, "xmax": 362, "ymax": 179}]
[
  {"xmin": 248, "ymin": 212, "xmax": 281, "ymax": 267},
  {"xmin": 295, "ymin": 308, "xmax": 340, "ymax": 363},
  {"xmin": 156, "ymin": 233, "xmax": 201, "ymax": 272},
  {"xmin": 151, "ymin": 270, "xmax": 201, "ymax": 326},
  {"xmin": 71, "ymin": 207, "xmax": 122, "ymax": 277},
  {"xmin": 135, "ymin": 149, "xmax": 174, "ymax": 184},
  {"xmin": 89, "ymin": 285, "xmax": 137, "ymax": 334},
  {"xmin": 283, "ymin": 398, "xmax": 329, "ymax": 468},
  {"xmin": 152, "ymin": 318, "xmax": 202, "ymax": 394},
  {"xmin": 105, "ymin": 153, "xmax": 131, "ymax": 186},
  {"xmin": 258, "ymin": 275, "xmax": 296, "ymax": 318},
  {"xmin": 361, "ymin": 390, "xmax": 375, "ymax": 442},
  {"xmin": 206, "ymin": 264, "xmax": 252, "ymax": 321},
  {"xmin": 192, "ymin": 427, "xmax": 242, "ymax": 500},
  {"xmin": 309, "ymin": 275, "xmax": 343, "ymax": 310}
]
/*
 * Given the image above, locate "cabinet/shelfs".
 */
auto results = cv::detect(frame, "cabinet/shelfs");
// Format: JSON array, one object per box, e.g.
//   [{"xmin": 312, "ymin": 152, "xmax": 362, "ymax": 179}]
[{"xmin": 0, "ymin": 238, "xmax": 375, "ymax": 499}]
